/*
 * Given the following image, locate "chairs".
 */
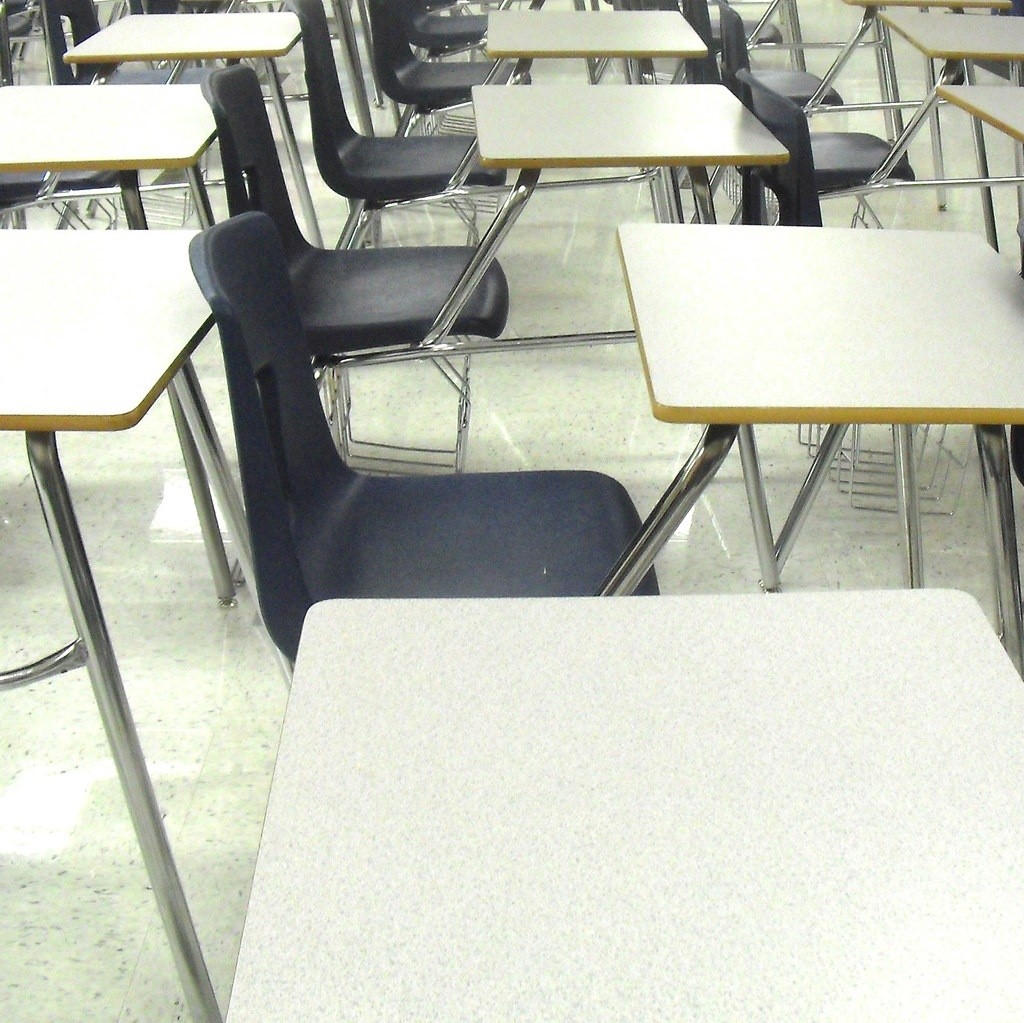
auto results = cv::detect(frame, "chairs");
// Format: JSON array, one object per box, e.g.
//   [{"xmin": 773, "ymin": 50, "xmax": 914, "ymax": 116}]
[
  {"xmin": 0, "ymin": 3, "xmax": 123, "ymax": 229},
  {"xmin": 658, "ymin": 0, "xmax": 783, "ymax": 84},
  {"xmin": 733, "ymin": 66, "xmax": 974, "ymax": 517},
  {"xmin": 367, "ymin": 0, "xmax": 516, "ymax": 138},
  {"xmin": 717, "ymin": 0, "xmax": 916, "ymax": 229},
  {"xmin": 187, "ymin": 211, "xmax": 660, "ymax": 664},
  {"xmin": 200, "ymin": 63, "xmax": 510, "ymax": 477},
  {"xmin": 682, "ymin": 0, "xmax": 843, "ymax": 207},
  {"xmin": 397, "ymin": 0, "xmax": 494, "ymax": 63},
  {"xmin": 285, "ymin": 0, "xmax": 507, "ymax": 250},
  {"xmin": 39, "ymin": 0, "xmax": 211, "ymax": 85}
]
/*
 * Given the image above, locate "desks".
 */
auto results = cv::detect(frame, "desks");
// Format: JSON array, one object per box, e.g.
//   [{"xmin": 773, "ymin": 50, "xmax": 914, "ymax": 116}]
[
  {"xmin": 0, "ymin": 227, "xmax": 294, "ymax": 1023},
  {"xmin": 937, "ymin": 84, "xmax": 1024, "ymax": 276},
  {"xmin": 227, "ymin": 589, "xmax": 1024, "ymax": 1023},
  {"xmin": 0, "ymin": 82, "xmax": 218, "ymax": 229},
  {"xmin": 444, "ymin": 9, "xmax": 708, "ymax": 195},
  {"xmin": 409, "ymin": 84, "xmax": 790, "ymax": 348},
  {"xmin": 595, "ymin": 223, "xmax": 1024, "ymax": 682},
  {"xmin": 861, "ymin": 11, "xmax": 1024, "ymax": 196},
  {"xmin": 804, "ymin": 0, "xmax": 1013, "ymax": 118},
  {"xmin": 62, "ymin": 12, "xmax": 324, "ymax": 249}
]
